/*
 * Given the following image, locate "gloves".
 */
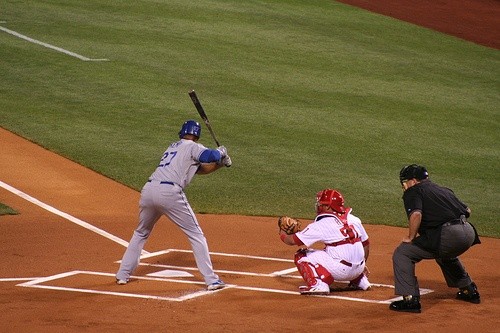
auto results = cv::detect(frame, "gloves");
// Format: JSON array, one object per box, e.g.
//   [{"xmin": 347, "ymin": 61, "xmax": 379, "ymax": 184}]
[
  {"xmin": 216, "ymin": 146, "xmax": 227, "ymax": 157},
  {"xmin": 220, "ymin": 155, "xmax": 232, "ymax": 167}
]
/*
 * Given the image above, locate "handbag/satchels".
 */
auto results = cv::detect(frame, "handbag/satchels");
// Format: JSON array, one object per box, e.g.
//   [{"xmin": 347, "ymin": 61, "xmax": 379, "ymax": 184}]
[
  {"xmin": 412, "ymin": 227, "xmax": 442, "ymax": 255},
  {"xmin": 468, "ymin": 221, "xmax": 483, "ymax": 248}
]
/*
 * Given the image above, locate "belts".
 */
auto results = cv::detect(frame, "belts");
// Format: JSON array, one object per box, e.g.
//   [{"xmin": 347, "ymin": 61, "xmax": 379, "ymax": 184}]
[
  {"xmin": 442, "ymin": 221, "xmax": 459, "ymax": 227},
  {"xmin": 147, "ymin": 180, "xmax": 173, "ymax": 185},
  {"xmin": 341, "ymin": 260, "xmax": 364, "ymax": 267}
]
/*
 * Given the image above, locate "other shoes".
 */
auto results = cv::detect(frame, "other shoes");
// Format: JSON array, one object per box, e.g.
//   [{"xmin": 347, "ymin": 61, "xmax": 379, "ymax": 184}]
[
  {"xmin": 116, "ymin": 279, "xmax": 129, "ymax": 284},
  {"xmin": 348, "ymin": 279, "xmax": 371, "ymax": 291},
  {"xmin": 299, "ymin": 282, "xmax": 330, "ymax": 294},
  {"xmin": 456, "ymin": 289, "xmax": 480, "ymax": 302},
  {"xmin": 208, "ymin": 279, "xmax": 225, "ymax": 289},
  {"xmin": 390, "ymin": 300, "xmax": 421, "ymax": 312}
]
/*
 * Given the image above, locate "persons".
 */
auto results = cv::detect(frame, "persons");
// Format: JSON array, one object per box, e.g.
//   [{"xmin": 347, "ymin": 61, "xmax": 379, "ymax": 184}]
[
  {"xmin": 115, "ymin": 120, "xmax": 231, "ymax": 291},
  {"xmin": 279, "ymin": 189, "xmax": 372, "ymax": 295},
  {"xmin": 390, "ymin": 164, "xmax": 482, "ymax": 313}
]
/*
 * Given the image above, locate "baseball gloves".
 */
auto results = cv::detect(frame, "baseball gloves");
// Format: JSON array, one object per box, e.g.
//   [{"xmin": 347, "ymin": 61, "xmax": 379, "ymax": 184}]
[{"xmin": 277, "ymin": 216, "xmax": 302, "ymax": 235}]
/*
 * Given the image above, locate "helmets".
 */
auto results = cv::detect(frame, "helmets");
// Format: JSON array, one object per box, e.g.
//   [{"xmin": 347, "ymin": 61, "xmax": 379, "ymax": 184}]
[
  {"xmin": 317, "ymin": 188, "xmax": 345, "ymax": 212},
  {"xmin": 178, "ymin": 120, "xmax": 201, "ymax": 138},
  {"xmin": 399, "ymin": 164, "xmax": 428, "ymax": 180}
]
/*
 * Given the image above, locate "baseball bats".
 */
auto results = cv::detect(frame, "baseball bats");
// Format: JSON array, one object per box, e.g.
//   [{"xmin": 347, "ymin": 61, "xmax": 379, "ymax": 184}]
[{"xmin": 188, "ymin": 90, "xmax": 231, "ymax": 168}]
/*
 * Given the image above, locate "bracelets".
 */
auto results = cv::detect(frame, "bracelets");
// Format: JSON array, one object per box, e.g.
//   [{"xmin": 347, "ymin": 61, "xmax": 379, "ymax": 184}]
[{"xmin": 280, "ymin": 233, "xmax": 288, "ymax": 242}]
